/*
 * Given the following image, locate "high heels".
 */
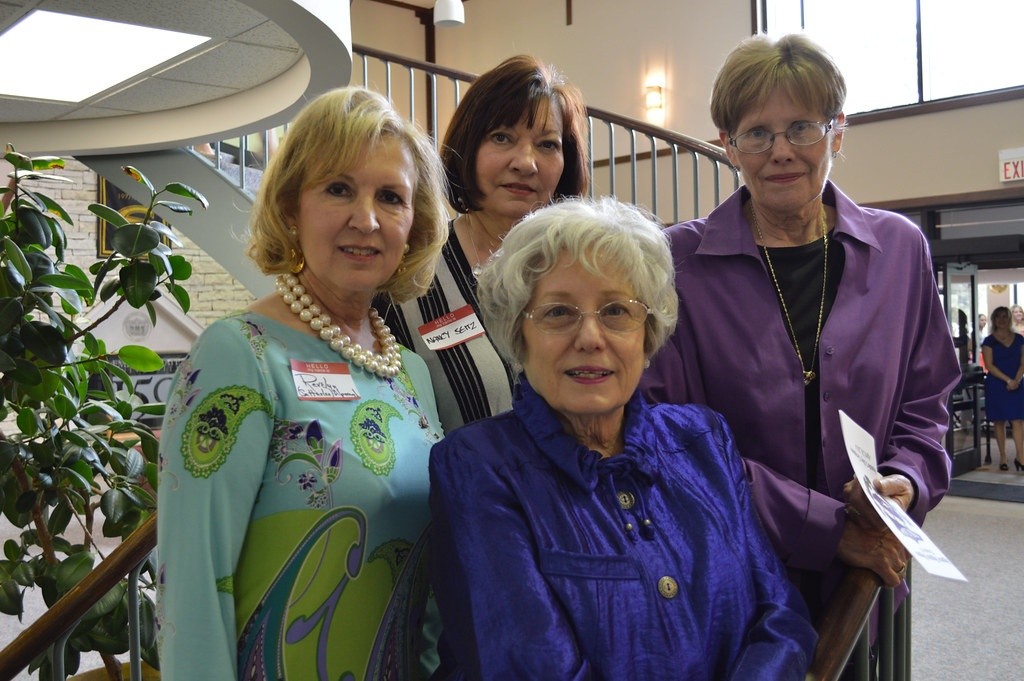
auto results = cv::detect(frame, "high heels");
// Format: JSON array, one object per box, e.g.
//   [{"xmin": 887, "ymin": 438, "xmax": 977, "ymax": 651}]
[
  {"xmin": 1014, "ymin": 458, "xmax": 1024, "ymax": 471},
  {"xmin": 1000, "ymin": 463, "xmax": 1009, "ymax": 470}
]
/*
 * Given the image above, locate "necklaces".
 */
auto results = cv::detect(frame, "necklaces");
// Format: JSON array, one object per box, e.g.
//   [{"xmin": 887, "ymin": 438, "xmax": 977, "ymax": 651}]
[
  {"xmin": 275, "ymin": 274, "xmax": 402, "ymax": 377},
  {"xmin": 750, "ymin": 200, "xmax": 828, "ymax": 386},
  {"xmin": 464, "ymin": 214, "xmax": 484, "ymax": 266}
]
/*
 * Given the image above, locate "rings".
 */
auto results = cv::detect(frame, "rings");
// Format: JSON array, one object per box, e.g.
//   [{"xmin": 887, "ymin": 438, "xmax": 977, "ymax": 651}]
[
  {"xmin": 898, "ymin": 562, "xmax": 906, "ymax": 573},
  {"xmin": 845, "ymin": 505, "xmax": 859, "ymax": 516}
]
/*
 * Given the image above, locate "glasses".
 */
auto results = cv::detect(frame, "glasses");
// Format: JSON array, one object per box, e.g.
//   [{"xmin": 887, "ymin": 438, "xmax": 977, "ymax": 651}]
[
  {"xmin": 522, "ymin": 299, "xmax": 657, "ymax": 335},
  {"xmin": 728, "ymin": 116, "xmax": 835, "ymax": 154}
]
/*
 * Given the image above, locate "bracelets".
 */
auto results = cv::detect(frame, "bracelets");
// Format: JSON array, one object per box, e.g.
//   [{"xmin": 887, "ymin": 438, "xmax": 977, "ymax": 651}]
[{"xmin": 1007, "ymin": 378, "xmax": 1013, "ymax": 384}]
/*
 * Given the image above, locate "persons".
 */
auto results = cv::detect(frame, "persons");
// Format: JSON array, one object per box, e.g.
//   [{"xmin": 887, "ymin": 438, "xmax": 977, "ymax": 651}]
[
  {"xmin": 429, "ymin": 197, "xmax": 820, "ymax": 681},
  {"xmin": 1010, "ymin": 304, "xmax": 1024, "ymax": 336},
  {"xmin": 636, "ymin": 33, "xmax": 963, "ymax": 681},
  {"xmin": 980, "ymin": 307, "xmax": 1024, "ymax": 472},
  {"xmin": 360, "ymin": 54, "xmax": 593, "ymax": 437},
  {"xmin": 970, "ymin": 314, "xmax": 988, "ymax": 374},
  {"xmin": 156, "ymin": 85, "xmax": 446, "ymax": 681}
]
